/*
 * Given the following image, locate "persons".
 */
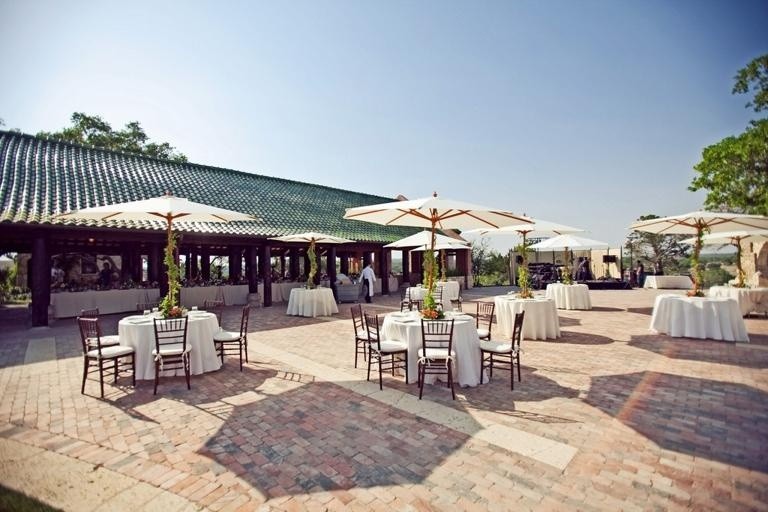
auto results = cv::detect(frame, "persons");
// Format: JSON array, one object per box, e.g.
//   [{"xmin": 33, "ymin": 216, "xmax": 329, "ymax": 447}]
[
  {"xmin": 579, "ymin": 256, "xmax": 590, "ymax": 283},
  {"xmin": 654, "ymin": 258, "xmax": 664, "ymax": 276},
  {"xmin": 356, "ymin": 262, "xmax": 379, "ymax": 301},
  {"xmin": 48, "ymin": 261, "xmax": 116, "ymax": 287},
  {"xmin": 635, "ymin": 260, "xmax": 645, "ymax": 288}
]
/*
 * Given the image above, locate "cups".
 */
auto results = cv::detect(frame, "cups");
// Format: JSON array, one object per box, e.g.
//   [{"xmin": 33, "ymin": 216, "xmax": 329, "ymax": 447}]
[
  {"xmin": 402, "ymin": 303, "xmax": 460, "ymax": 319},
  {"xmin": 141, "ymin": 306, "xmax": 199, "ymax": 317}
]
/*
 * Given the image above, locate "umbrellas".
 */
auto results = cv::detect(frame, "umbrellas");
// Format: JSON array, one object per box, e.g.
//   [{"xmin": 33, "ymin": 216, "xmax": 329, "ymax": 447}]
[
  {"xmin": 381, "ymin": 228, "xmax": 468, "ymax": 283},
  {"xmin": 467, "ymin": 212, "xmax": 586, "ymax": 297},
  {"xmin": 342, "ymin": 193, "xmax": 535, "ymax": 309},
  {"xmin": 526, "ymin": 232, "xmax": 610, "ymax": 286},
  {"xmin": 49, "ymin": 191, "xmax": 264, "ymax": 315},
  {"xmin": 268, "ymin": 231, "xmax": 357, "ymax": 288},
  {"xmin": 629, "ymin": 207, "xmax": 767, "ymax": 286},
  {"xmin": 408, "ymin": 241, "xmax": 474, "ymax": 282},
  {"xmin": 680, "ymin": 229, "xmax": 766, "ymax": 287}
]
